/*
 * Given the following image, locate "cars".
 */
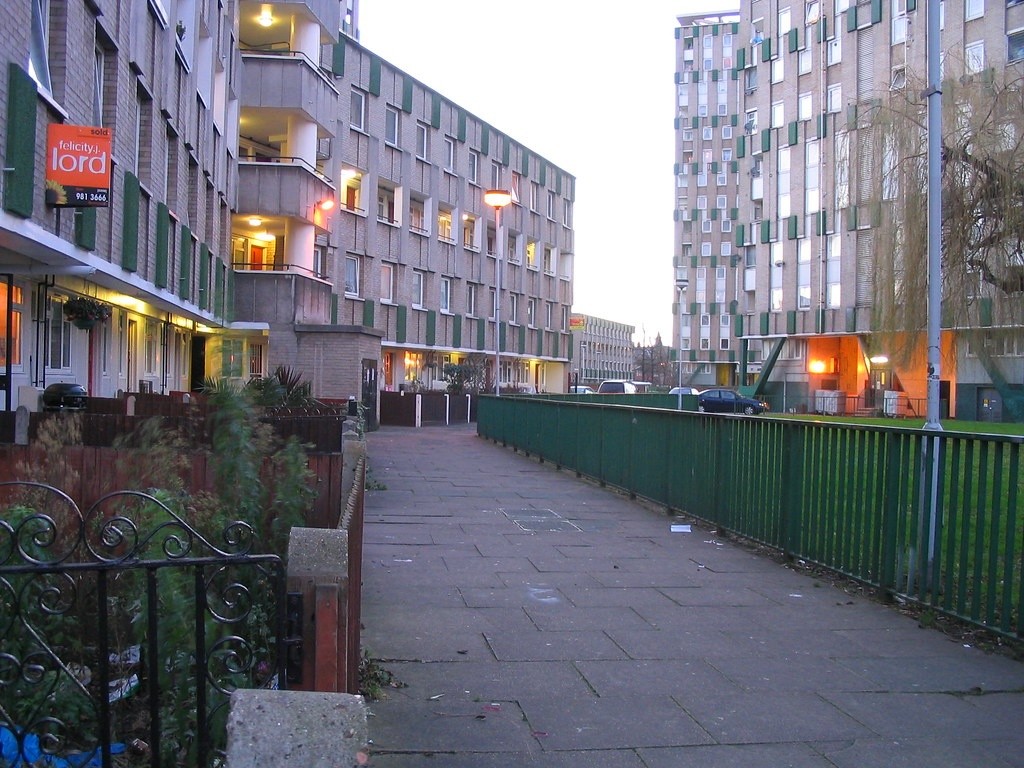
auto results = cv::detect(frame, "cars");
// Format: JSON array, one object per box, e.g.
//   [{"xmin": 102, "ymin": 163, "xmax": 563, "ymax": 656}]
[
  {"xmin": 598, "ymin": 380, "xmax": 640, "ymax": 394},
  {"xmin": 668, "ymin": 386, "xmax": 699, "ymax": 394},
  {"xmin": 698, "ymin": 389, "xmax": 769, "ymax": 416},
  {"xmin": 570, "ymin": 385, "xmax": 593, "ymax": 394}
]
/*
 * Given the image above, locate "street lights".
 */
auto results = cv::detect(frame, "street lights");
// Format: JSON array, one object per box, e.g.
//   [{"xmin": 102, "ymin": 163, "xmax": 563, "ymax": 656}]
[
  {"xmin": 485, "ymin": 189, "xmax": 512, "ymax": 395},
  {"xmin": 677, "ymin": 279, "xmax": 687, "ymax": 409}
]
